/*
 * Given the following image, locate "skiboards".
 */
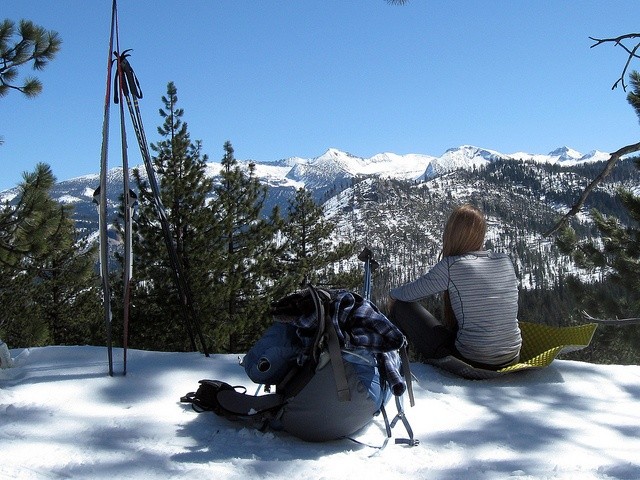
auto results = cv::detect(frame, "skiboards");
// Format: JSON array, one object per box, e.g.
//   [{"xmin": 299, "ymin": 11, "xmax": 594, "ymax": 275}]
[{"xmin": 92, "ymin": 0, "xmax": 134, "ymax": 377}]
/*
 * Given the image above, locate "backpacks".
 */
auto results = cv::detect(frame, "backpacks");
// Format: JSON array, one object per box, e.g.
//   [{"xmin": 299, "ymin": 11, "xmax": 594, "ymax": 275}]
[{"xmin": 181, "ymin": 324, "xmax": 422, "ymax": 447}]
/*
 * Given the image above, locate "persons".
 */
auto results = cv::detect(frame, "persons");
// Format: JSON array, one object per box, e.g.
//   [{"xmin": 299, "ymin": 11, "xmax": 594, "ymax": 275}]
[{"xmin": 387, "ymin": 207, "xmax": 522, "ymax": 370}]
